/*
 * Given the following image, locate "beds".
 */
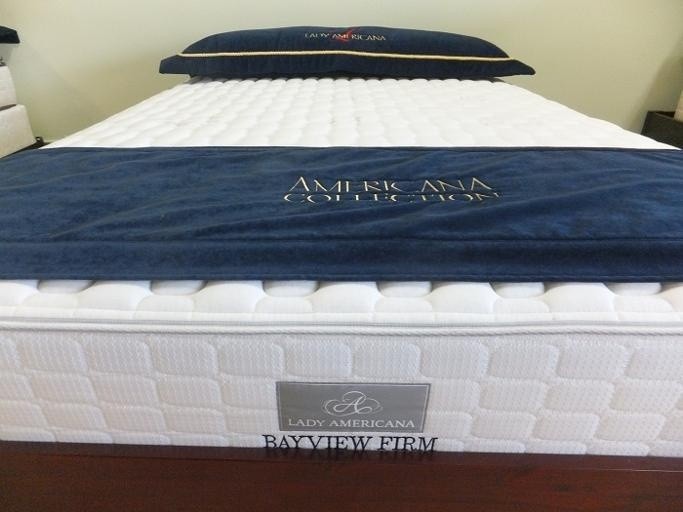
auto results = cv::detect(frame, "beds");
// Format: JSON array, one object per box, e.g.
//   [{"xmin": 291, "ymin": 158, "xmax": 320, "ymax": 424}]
[{"xmin": 0, "ymin": 78, "xmax": 683, "ymax": 512}]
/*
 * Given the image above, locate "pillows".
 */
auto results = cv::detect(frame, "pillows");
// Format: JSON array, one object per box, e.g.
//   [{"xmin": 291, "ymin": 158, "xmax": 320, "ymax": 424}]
[{"xmin": 159, "ymin": 26, "xmax": 535, "ymax": 77}]
